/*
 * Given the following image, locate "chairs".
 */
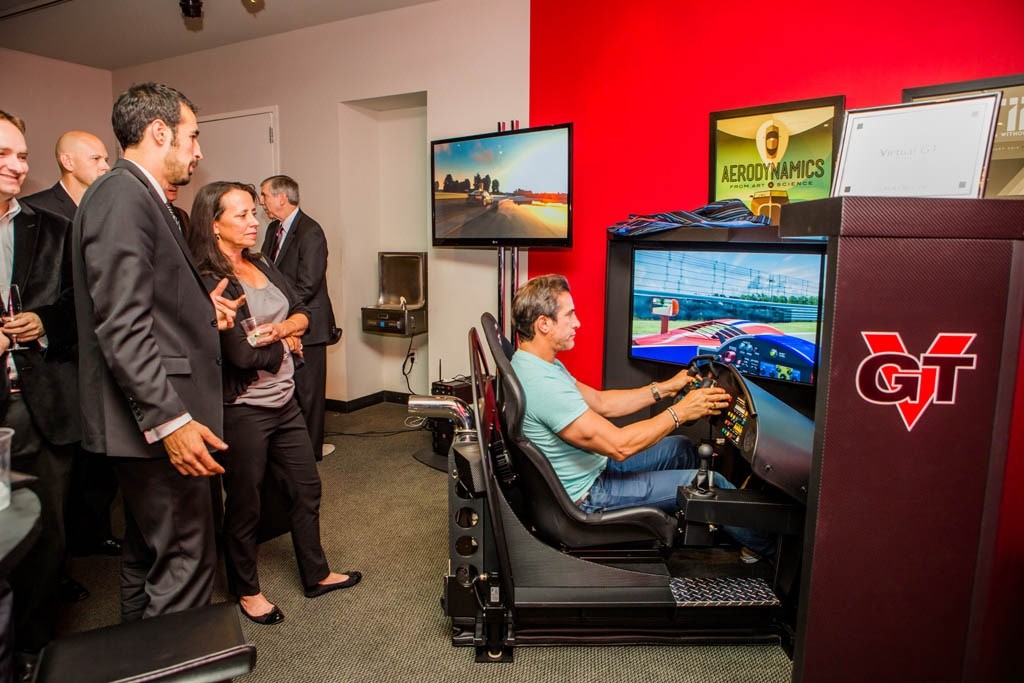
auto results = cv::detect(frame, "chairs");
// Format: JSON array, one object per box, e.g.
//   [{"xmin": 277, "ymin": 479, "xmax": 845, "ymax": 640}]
[{"xmin": 482, "ymin": 313, "xmax": 670, "ymax": 555}]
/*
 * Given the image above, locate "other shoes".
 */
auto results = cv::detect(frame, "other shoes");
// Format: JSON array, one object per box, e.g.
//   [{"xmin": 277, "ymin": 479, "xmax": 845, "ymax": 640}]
[
  {"xmin": 740, "ymin": 544, "xmax": 776, "ymax": 564},
  {"xmin": 304, "ymin": 570, "xmax": 361, "ymax": 598},
  {"xmin": 102, "ymin": 537, "xmax": 125, "ymax": 555},
  {"xmin": 238, "ymin": 593, "xmax": 284, "ymax": 625},
  {"xmin": 59, "ymin": 574, "xmax": 90, "ymax": 601}
]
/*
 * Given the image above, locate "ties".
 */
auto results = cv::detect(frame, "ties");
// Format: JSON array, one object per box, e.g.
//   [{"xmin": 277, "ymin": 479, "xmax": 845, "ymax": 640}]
[{"xmin": 270, "ymin": 225, "xmax": 283, "ymax": 263}]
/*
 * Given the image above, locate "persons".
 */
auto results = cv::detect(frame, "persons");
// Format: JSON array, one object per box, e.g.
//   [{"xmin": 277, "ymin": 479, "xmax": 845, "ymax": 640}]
[
  {"xmin": 0, "ymin": 82, "xmax": 364, "ymax": 683},
  {"xmin": 508, "ymin": 271, "xmax": 774, "ymax": 565}
]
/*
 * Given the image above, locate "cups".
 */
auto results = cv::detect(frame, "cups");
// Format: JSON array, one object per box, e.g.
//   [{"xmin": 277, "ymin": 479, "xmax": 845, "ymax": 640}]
[
  {"xmin": 0, "ymin": 428, "xmax": 15, "ymax": 510},
  {"xmin": 240, "ymin": 315, "xmax": 270, "ymax": 347}
]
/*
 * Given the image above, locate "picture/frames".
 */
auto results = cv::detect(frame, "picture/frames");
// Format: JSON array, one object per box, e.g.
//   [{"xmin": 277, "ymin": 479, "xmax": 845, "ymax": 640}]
[
  {"xmin": 904, "ymin": 75, "xmax": 1023, "ymax": 198},
  {"xmin": 708, "ymin": 95, "xmax": 845, "ymax": 220}
]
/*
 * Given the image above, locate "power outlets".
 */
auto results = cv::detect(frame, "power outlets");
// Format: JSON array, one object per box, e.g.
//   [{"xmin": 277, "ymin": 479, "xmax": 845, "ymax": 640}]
[{"xmin": 409, "ymin": 350, "xmax": 417, "ymax": 363}]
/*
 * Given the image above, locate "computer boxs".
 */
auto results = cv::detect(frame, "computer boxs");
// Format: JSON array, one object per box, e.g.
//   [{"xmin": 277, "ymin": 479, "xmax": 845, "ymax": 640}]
[{"xmin": 431, "ymin": 374, "xmax": 498, "ymax": 457}]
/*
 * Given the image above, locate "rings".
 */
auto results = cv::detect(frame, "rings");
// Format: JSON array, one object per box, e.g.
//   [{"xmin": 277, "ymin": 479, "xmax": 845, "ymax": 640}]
[{"xmin": 712, "ymin": 403, "xmax": 717, "ymax": 409}]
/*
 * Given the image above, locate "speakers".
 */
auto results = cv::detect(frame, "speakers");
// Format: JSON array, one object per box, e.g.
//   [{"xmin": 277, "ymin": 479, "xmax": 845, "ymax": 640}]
[{"xmin": 650, "ymin": 361, "xmax": 721, "ymax": 445}]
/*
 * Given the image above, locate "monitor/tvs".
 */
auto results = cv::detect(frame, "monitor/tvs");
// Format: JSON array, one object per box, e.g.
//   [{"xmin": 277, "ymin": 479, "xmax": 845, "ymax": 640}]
[
  {"xmin": 627, "ymin": 244, "xmax": 825, "ymax": 387},
  {"xmin": 430, "ymin": 121, "xmax": 573, "ymax": 248}
]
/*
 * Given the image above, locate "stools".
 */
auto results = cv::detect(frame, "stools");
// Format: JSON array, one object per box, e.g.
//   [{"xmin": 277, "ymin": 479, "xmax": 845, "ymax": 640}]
[{"xmin": 36, "ymin": 599, "xmax": 257, "ymax": 683}]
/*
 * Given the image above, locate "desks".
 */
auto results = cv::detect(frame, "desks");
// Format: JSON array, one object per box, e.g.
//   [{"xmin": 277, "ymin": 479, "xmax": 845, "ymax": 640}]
[{"xmin": 1, "ymin": 486, "xmax": 47, "ymax": 596}]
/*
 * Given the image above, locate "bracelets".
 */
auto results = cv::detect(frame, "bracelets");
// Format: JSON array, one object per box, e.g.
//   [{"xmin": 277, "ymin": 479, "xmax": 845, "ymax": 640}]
[
  {"xmin": 282, "ymin": 319, "xmax": 298, "ymax": 332},
  {"xmin": 649, "ymin": 381, "xmax": 662, "ymax": 401},
  {"xmin": 292, "ymin": 336, "xmax": 298, "ymax": 351},
  {"xmin": 667, "ymin": 406, "xmax": 680, "ymax": 429}
]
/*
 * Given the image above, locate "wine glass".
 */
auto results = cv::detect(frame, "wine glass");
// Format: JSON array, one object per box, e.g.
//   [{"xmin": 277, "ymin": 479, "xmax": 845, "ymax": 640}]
[{"xmin": 0, "ymin": 285, "xmax": 30, "ymax": 351}]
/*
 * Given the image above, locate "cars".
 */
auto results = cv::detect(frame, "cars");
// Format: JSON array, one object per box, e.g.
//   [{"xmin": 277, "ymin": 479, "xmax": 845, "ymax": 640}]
[{"xmin": 465, "ymin": 190, "xmax": 492, "ymax": 207}]
